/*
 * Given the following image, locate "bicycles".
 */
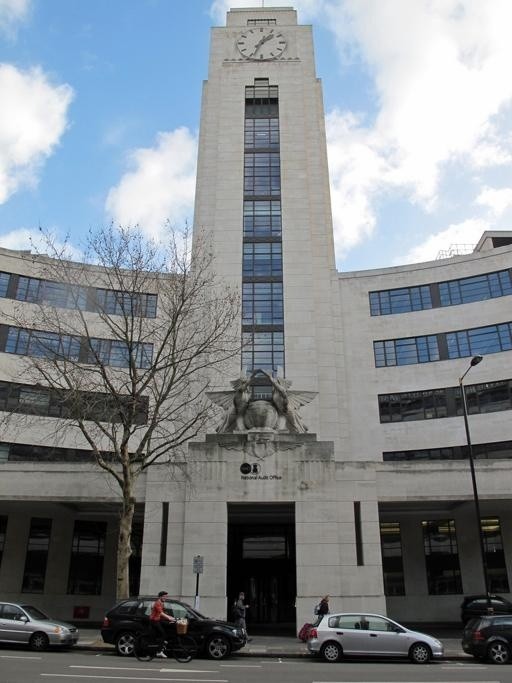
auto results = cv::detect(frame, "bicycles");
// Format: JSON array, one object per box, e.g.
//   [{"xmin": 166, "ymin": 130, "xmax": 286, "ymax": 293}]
[{"xmin": 135, "ymin": 616, "xmax": 197, "ymax": 663}]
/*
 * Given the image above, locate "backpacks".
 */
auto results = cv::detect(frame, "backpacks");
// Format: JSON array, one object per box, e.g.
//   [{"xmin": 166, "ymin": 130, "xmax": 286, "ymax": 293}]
[{"xmin": 314, "ymin": 603, "xmax": 322, "ymax": 615}]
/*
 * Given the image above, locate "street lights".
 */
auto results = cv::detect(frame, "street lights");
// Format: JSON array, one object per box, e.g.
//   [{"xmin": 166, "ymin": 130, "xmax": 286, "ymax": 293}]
[{"xmin": 459, "ymin": 354, "xmax": 494, "ymax": 616}]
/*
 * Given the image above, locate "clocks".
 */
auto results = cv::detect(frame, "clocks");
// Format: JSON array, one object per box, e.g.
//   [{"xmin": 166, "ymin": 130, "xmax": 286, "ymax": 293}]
[{"xmin": 235, "ymin": 26, "xmax": 287, "ymax": 62}]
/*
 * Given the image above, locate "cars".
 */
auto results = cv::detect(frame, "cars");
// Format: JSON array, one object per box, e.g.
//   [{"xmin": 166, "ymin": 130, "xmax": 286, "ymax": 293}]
[
  {"xmin": 307, "ymin": 612, "xmax": 444, "ymax": 664},
  {"xmin": 461, "ymin": 615, "xmax": 512, "ymax": 664},
  {"xmin": 0, "ymin": 602, "xmax": 79, "ymax": 652}
]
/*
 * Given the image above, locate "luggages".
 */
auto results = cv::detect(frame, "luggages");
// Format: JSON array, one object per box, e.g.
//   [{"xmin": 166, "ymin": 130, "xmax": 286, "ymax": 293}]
[{"xmin": 299, "ymin": 619, "xmax": 322, "ymax": 643}]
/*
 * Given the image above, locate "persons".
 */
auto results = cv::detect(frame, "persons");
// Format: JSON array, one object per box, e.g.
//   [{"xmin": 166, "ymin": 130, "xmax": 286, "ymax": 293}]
[
  {"xmin": 312, "ymin": 592, "xmax": 332, "ymax": 625},
  {"xmin": 146, "ymin": 589, "xmax": 176, "ymax": 658},
  {"xmin": 231, "ymin": 590, "xmax": 255, "ymax": 641}
]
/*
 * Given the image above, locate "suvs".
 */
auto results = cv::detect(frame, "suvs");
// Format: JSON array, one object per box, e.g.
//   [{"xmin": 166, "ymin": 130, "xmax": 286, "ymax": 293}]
[{"xmin": 101, "ymin": 595, "xmax": 247, "ymax": 659}]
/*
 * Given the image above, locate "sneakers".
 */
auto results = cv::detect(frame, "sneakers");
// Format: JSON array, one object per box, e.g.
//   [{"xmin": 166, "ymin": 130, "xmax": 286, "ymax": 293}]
[{"xmin": 156, "ymin": 640, "xmax": 168, "ymax": 658}]
